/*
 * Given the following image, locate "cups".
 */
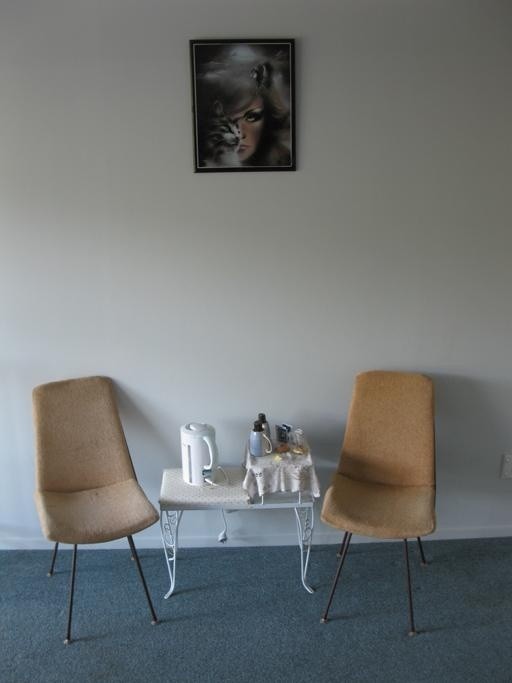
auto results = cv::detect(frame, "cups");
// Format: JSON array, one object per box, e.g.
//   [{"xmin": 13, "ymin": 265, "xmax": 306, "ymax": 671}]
[{"xmin": 249, "ymin": 413, "xmax": 273, "ymax": 457}]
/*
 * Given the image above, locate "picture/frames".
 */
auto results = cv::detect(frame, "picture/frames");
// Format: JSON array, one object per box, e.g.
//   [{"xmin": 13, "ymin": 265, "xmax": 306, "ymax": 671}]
[{"xmin": 189, "ymin": 37, "xmax": 296, "ymax": 172}]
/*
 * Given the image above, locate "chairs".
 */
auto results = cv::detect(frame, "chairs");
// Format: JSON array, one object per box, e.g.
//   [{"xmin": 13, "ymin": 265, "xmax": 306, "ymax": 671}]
[
  {"xmin": 31, "ymin": 374, "xmax": 160, "ymax": 647},
  {"xmin": 319, "ymin": 368, "xmax": 438, "ymax": 638}
]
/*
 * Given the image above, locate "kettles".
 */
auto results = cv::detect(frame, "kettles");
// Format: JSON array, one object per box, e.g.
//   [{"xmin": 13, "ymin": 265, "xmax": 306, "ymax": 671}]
[{"xmin": 181, "ymin": 420, "xmax": 218, "ymax": 487}]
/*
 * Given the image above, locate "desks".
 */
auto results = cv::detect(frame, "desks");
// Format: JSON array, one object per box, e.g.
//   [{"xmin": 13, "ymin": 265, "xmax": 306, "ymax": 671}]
[{"xmin": 157, "ymin": 467, "xmax": 322, "ymax": 600}]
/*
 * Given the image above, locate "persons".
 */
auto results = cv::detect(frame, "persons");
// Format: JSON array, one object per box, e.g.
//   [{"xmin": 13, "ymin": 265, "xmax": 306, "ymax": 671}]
[{"xmin": 197, "ymin": 44, "xmax": 291, "ymax": 166}]
